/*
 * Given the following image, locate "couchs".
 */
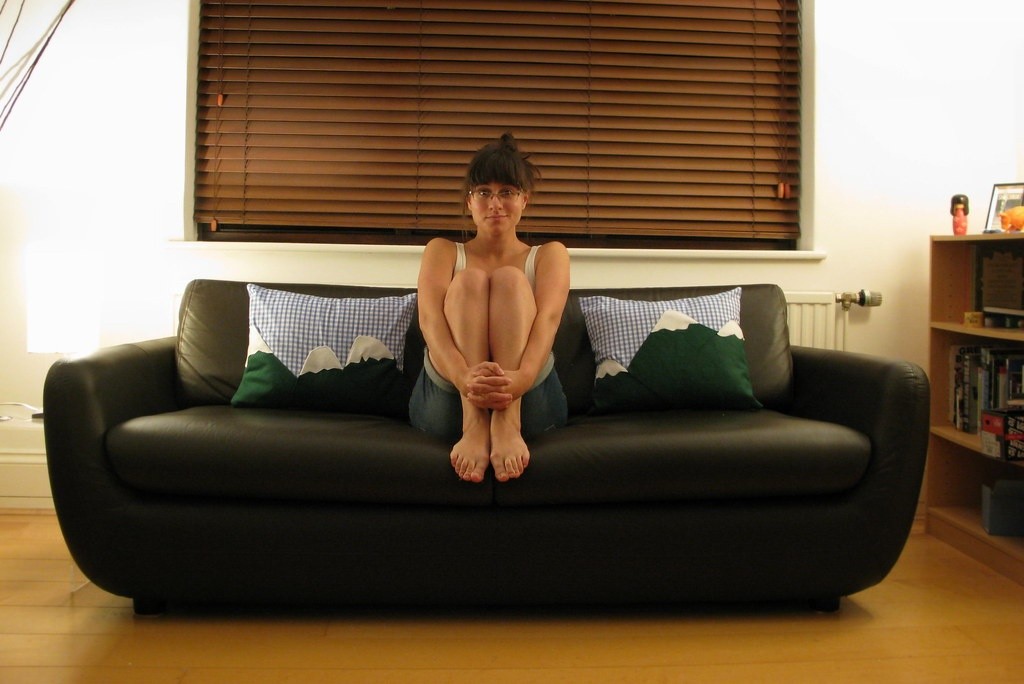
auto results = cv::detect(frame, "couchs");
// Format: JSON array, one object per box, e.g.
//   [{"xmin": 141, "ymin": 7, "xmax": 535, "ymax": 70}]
[{"xmin": 42, "ymin": 278, "xmax": 933, "ymax": 617}]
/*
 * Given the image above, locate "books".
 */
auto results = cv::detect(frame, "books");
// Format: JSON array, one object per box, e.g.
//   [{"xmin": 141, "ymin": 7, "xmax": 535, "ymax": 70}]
[{"xmin": 946, "ymin": 342, "xmax": 1024, "ymax": 440}]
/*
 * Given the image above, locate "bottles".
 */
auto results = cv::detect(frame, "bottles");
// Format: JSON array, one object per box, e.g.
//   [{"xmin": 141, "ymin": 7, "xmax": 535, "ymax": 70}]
[{"xmin": 950, "ymin": 195, "xmax": 969, "ymax": 236}]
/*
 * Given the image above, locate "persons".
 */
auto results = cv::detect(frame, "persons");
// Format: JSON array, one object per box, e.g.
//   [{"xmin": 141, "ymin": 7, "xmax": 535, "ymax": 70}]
[{"xmin": 408, "ymin": 132, "xmax": 571, "ymax": 483}]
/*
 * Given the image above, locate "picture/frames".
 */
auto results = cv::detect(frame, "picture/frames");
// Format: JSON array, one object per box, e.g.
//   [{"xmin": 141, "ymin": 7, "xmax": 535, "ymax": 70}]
[{"xmin": 986, "ymin": 182, "xmax": 1024, "ymax": 233}]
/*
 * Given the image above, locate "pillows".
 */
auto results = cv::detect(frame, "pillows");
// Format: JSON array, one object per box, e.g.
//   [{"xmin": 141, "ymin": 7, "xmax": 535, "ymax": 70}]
[
  {"xmin": 578, "ymin": 286, "xmax": 763, "ymax": 417},
  {"xmin": 231, "ymin": 284, "xmax": 418, "ymax": 417}
]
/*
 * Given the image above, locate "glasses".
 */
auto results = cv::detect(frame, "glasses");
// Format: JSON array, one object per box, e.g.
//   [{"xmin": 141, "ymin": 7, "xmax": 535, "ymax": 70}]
[{"xmin": 468, "ymin": 186, "xmax": 522, "ymax": 202}]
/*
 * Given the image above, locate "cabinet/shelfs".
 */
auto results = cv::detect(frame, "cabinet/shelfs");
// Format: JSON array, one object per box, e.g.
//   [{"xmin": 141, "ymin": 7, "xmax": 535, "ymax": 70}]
[{"xmin": 924, "ymin": 234, "xmax": 1023, "ymax": 584}]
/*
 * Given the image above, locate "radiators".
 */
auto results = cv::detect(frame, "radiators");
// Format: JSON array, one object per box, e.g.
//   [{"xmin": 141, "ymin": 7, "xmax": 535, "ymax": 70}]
[{"xmin": 784, "ymin": 289, "xmax": 883, "ymax": 350}]
